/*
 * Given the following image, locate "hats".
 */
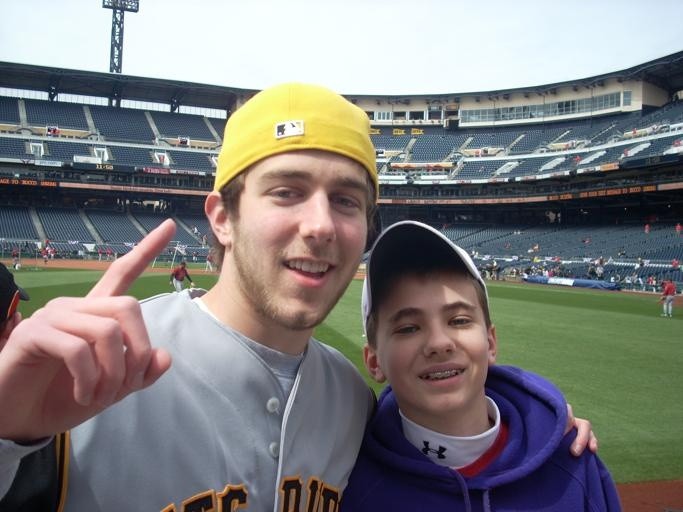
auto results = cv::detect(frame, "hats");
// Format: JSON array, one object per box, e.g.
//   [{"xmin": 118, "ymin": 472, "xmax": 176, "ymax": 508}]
[
  {"xmin": 212, "ymin": 82, "xmax": 379, "ymax": 205},
  {"xmin": 0, "ymin": 261, "xmax": 31, "ymax": 323},
  {"xmin": 360, "ymin": 219, "xmax": 489, "ymax": 345}
]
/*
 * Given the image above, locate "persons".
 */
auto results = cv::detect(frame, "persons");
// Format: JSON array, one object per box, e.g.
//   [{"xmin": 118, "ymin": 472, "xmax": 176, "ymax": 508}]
[
  {"xmin": 167, "ymin": 260, "xmax": 195, "ymax": 293},
  {"xmin": 472, "ymin": 250, "xmax": 679, "ymax": 295},
  {"xmin": 0, "ymin": 82, "xmax": 602, "ymax": 510},
  {"xmin": 657, "ymin": 277, "xmax": 676, "ymax": 318},
  {"xmin": 0, "ymin": 259, "xmax": 30, "ymax": 352},
  {"xmin": 0, "ymin": 224, "xmax": 216, "ymax": 274},
  {"xmin": 338, "ymin": 215, "xmax": 624, "ymax": 511}
]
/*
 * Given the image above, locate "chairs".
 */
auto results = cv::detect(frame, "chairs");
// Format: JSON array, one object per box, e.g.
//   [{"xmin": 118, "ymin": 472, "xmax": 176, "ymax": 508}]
[{"xmin": 0, "ymin": 86, "xmax": 683, "ymax": 298}]
